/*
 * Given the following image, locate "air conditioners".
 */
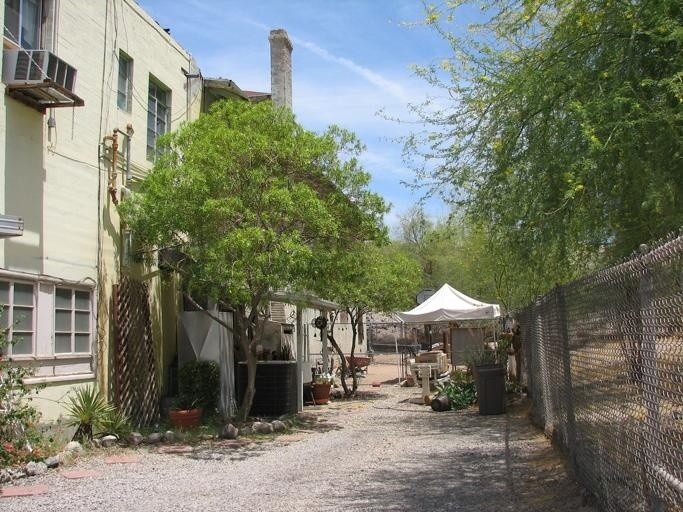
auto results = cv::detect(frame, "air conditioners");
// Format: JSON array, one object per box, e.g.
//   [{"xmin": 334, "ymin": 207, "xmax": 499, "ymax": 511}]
[{"xmin": 2, "ymin": 49, "xmax": 77, "ymax": 101}]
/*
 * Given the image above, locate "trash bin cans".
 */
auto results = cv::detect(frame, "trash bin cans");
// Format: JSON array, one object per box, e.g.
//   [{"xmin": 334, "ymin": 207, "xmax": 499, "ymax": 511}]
[{"xmin": 473, "ymin": 364, "xmax": 507, "ymax": 415}]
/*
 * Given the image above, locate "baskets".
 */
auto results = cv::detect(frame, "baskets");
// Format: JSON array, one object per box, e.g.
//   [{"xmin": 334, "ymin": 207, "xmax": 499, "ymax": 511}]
[
  {"xmin": 346, "ymin": 356, "xmax": 371, "ymax": 367},
  {"xmin": 303, "ymin": 382, "xmax": 331, "ymax": 403}
]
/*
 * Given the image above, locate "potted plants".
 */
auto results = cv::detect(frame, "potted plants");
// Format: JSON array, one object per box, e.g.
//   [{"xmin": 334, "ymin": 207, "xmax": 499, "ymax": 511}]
[
  {"xmin": 311, "ymin": 377, "xmax": 333, "ymax": 404},
  {"xmin": 431, "ymin": 384, "xmax": 477, "ymax": 412},
  {"xmin": 169, "ymin": 359, "xmax": 221, "ymax": 431}
]
